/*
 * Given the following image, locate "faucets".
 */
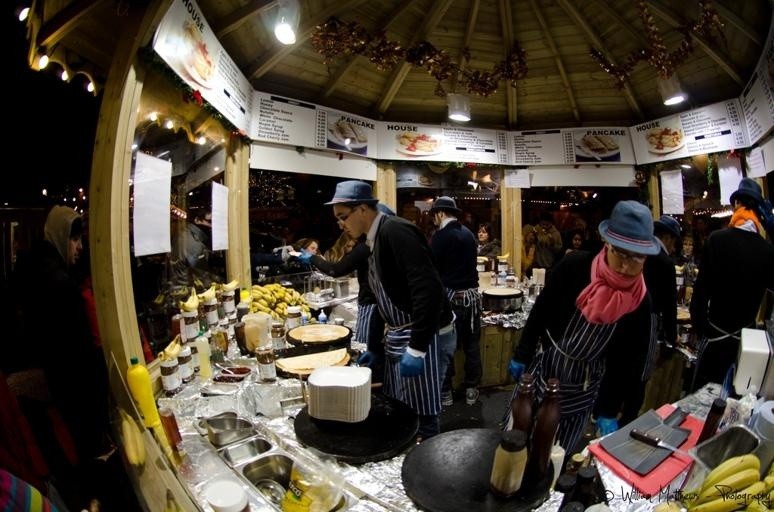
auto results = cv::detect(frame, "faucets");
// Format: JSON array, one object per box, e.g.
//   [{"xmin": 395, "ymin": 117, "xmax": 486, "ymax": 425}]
[{"xmin": 491, "ymin": 272, "xmax": 499, "ymax": 286}]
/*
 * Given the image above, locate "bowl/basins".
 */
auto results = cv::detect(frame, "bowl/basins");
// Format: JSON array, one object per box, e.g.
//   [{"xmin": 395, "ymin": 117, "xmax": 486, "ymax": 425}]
[{"xmin": 207, "ymin": 475, "xmax": 247, "ymax": 510}]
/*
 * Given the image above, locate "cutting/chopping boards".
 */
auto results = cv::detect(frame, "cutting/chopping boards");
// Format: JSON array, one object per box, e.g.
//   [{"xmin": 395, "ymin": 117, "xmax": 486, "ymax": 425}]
[{"xmin": 586, "ymin": 402, "xmax": 722, "ymax": 497}]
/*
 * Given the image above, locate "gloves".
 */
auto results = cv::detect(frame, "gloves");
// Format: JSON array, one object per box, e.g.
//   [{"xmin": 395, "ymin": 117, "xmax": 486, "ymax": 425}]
[
  {"xmin": 358, "ymin": 351, "xmax": 376, "ymax": 367},
  {"xmin": 399, "ymin": 352, "xmax": 423, "ymax": 377},
  {"xmin": 295, "ymin": 247, "xmax": 313, "ymax": 265},
  {"xmin": 593, "ymin": 416, "xmax": 618, "ymax": 436},
  {"xmin": 508, "ymin": 359, "xmax": 525, "ymax": 382}
]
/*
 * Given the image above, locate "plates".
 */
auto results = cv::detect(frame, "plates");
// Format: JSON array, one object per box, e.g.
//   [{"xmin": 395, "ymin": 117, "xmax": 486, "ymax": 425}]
[
  {"xmin": 574, "ymin": 129, "xmax": 619, "ymax": 159},
  {"xmin": 646, "ymin": 128, "xmax": 685, "ymax": 155},
  {"xmin": 395, "ymin": 144, "xmax": 441, "ymax": 156},
  {"xmin": 327, "ymin": 116, "xmax": 369, "ymax": 149},
  {"xmin": 306, "ymin": 364, "xmax": 371, "ymax": 425},
  {"xmin": 181, "ymin": 49, "xmax": 221, "ymax": 89}
]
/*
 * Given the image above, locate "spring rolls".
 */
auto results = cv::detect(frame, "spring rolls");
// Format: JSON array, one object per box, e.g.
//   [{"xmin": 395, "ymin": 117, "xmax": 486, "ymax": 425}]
[
  {"xmin": 336, "ymin": 119, "xmax": 368, "ymax": 144},
  {"xmin": 398, "ymin": 130, "xmax": 438, "ymax": 151},
  {"xmin": 647, "ymin": 128, "xmax": 681, "ymax": 147},
  {"xmin": 183, "ymin": 24, "xmax": 215, "ymax": 81},
  {"xmin": 582, "ymin": 134, "xmax": 618, "ymax": 153}
]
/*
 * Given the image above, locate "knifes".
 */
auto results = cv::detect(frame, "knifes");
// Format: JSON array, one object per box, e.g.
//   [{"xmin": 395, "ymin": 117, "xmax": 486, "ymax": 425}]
[{"xmin": 631, "ymin": 431, "xmax": 694, "ymax": 460}]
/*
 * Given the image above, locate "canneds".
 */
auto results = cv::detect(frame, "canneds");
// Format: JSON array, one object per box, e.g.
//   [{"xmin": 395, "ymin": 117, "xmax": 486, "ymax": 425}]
[
  {"xmin": 271, "ymin": 323, "xmax": 286, "ymax": 349},
  {"xmin": 160, "ymin": 290, "xmax": 239, "ymax": 396},
  {"xmin": 255, "ymin": 348, "xmax": 276, "ymax": 382},
  {"xmin": 681, "ymin": 324, "xmax": 692, "ymax": 343},
  {"xmin": 676, "ymin": 271, "xmax": 685, "ymax": 285},
  {"xmin": 476, "ymin": 259, "xmax": 516, "ymax": 286}
]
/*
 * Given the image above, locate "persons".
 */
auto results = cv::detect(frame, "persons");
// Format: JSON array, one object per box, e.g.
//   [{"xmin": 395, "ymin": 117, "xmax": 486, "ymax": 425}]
[
  {"xmin": 9, "ymin": 204, "xmax": 120, "ymax": 458},
  {"xmin": 177, "ymin": 176, "xmax": 774, "ymax": 410},
  {"xmin": 502, "ymin": 198, "xmax": 661, "ymax": 452},
  {"xmin": 323, "ymin": 180, "xmax": 458, "ymax": 441}
]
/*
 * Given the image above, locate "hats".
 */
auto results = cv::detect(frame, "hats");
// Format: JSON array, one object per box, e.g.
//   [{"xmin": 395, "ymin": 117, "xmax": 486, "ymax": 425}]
[
  {"xmin": 431, "ymin": 196, "xmax": 463, "ymax": 212},
  {"xmin": 598, "ymin": 200, "xmax": 662, "ymax": 255},
  {"xmin": 654, "ymin": 215, "xmax": 683, "ymax": 250},
  {"xmin": 323, "ymin": 181, "xmax": 379, "ymax": 205},
  {"xmin": 730, "ymin": 177, "xmax": 765, "ymax": 207}
]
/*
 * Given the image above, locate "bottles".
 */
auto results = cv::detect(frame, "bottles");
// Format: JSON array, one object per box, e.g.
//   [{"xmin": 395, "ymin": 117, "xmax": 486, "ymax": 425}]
[
  {"xmin": 125, "ymin": 288, "xmax": 344, "ymax": 458},
  {"xmin": 486, "ymin": 372, "xmax": 597, "ymax": 512},
  {"xmin": 694, "ymin": 397, "xmax": 728, "ymax": 449}
]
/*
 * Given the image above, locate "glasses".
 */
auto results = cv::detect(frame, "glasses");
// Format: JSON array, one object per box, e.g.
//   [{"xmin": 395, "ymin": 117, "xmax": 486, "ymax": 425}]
[
  {"xmin": 608, "ymin": 244, "xmax": 647, "ymax": 264},
  {"xmin": 337, "ymin": 209, "xmax": 355, "ymax": 224}
]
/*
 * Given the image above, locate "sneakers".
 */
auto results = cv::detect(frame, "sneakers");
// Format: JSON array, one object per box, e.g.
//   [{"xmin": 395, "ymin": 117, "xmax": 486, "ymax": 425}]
[
  {"xmin": 442, "ymin": 390, "xmax": 453, "ymax": 406},
  {"xmin": 466, "ymin": 387, "xmax": 480, "ymax": 404}
]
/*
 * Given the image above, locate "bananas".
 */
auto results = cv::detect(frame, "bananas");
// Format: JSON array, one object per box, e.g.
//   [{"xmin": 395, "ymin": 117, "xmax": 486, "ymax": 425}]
[
  {"xmin": 196, "ymin": 283, "xmax": 216, "ymax": 302},
  {"xmin": 497, "ymin": 252, "xmax": 509, "ymax": 259},
  {"xmin": 219, "ymin": 280, "xmax": 239, "ymax": 291},
  {"xmin": 192, "ymin": 275, "xmax": 203, "ymax": 287},
  {"xmin": 251, "ymin": 283, "xmax": 316, "ymax": 324},
  {"xmin": 653, "ymin": 454, "xmax": 773, "ymax": 512},
  {"xmin": 477, "ymin": 257, "xmax": 488, "ymax": 261},
  {"xmin": 170, "ymin": 285, "xmax": 189, "ymax": 295},
  {"xmin": 165, "ymin": 488, "xmax": 183, "ymax": 511},
  {"xmin": 152, "ymin": 294, "xmax": 164, "ymax": 304},
  {"xmin": 118, "ymin": 406, "xmax": 146, "ymax": 468}
]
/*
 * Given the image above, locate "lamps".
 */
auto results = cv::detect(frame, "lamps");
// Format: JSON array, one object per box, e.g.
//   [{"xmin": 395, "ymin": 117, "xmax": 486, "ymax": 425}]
[
  {"xmin": 274, "ymin": 0, "xmax": 301, "ymax": 45},
  {"xmin": 657, "ymin": 72, "xmax": 686, "ymax": 106},
  {"xmin": 445, "ymin": 91, "xmax": 472, "ymax": 122}
]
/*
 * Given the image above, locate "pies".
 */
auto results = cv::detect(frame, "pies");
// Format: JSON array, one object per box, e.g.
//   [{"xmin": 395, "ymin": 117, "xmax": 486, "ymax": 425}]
[
  {"xmin": 276, "ymin": 347, "xmax": 351, "ymax": 375},
  {"xmin": 676, "ymin": 310, "xmax": 691, "ymax": 319},
  {"xmin": 484, "ymin": 288, "xmax": 522, "ymax": 296},
  {"xmin": 289, "ymin": 324, "xmax": 350, "ymax": 342}
]
